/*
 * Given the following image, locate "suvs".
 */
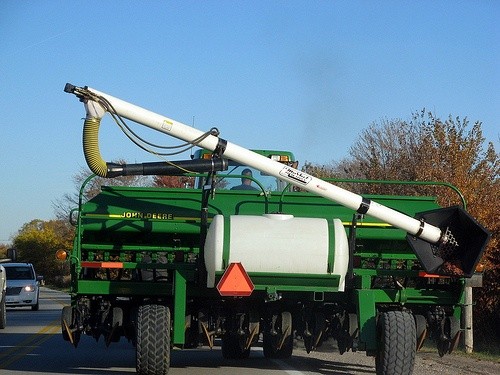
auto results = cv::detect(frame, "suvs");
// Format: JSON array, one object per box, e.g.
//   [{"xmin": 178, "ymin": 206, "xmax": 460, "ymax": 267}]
[{"xmin": 0, "ymin": 263, "xmax": 44, "ymax": 312}]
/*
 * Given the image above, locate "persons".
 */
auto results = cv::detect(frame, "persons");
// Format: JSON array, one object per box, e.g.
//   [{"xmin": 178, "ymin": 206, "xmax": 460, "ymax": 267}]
[{"xmin": 230, "ymin": 169, "xmax": 259, "ymax": 191}]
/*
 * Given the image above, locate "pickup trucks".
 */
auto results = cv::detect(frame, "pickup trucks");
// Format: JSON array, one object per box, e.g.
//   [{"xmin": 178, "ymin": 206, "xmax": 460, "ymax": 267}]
[{"xmin": 0, "ymin": 264, "xmax": 7, "ymax": 330}]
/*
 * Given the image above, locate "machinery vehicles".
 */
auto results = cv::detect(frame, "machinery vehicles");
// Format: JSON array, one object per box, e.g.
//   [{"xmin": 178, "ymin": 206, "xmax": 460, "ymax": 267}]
[{"xmin": 60, "ymin": 81, "xmax": 492, "ymax": 375}]
